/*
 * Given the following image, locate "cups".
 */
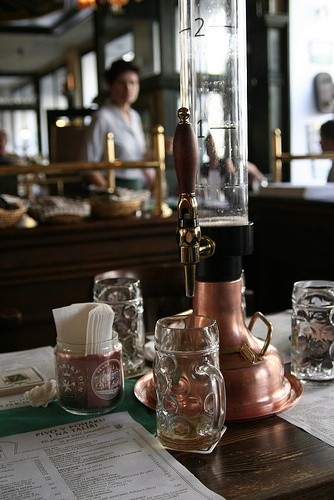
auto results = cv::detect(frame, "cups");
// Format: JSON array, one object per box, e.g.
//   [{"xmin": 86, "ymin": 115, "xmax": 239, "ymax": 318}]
[
  {"xmin": 93, "ymin": 277, "xmax": 146, "ymax": 378},
  {"xmin": 290, "ymin": 280, "xmax": 334, "ymax": 382},
  {"xmin": 154, "ymin": 315, "xmax": 226, "ymax": 450}
]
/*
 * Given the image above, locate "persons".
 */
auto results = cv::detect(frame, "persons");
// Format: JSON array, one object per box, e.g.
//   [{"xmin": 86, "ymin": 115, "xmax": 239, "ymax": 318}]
[
  {"xmin": 320, "ymin": 120, "xmax": 334, "ymax": 183},
  {"xmin": 83, "ymin": 60, "xmax": 154, "ymax": 191},
  {"xmin": 1, "ymin": 130, "xmax": 31, "ymax": 196}
]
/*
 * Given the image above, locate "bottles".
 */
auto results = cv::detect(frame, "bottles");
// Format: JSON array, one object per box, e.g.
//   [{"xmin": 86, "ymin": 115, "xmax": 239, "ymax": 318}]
[{"xmin": 55, "ymin": 332, "xmax": 124, "ymax": 416}]
